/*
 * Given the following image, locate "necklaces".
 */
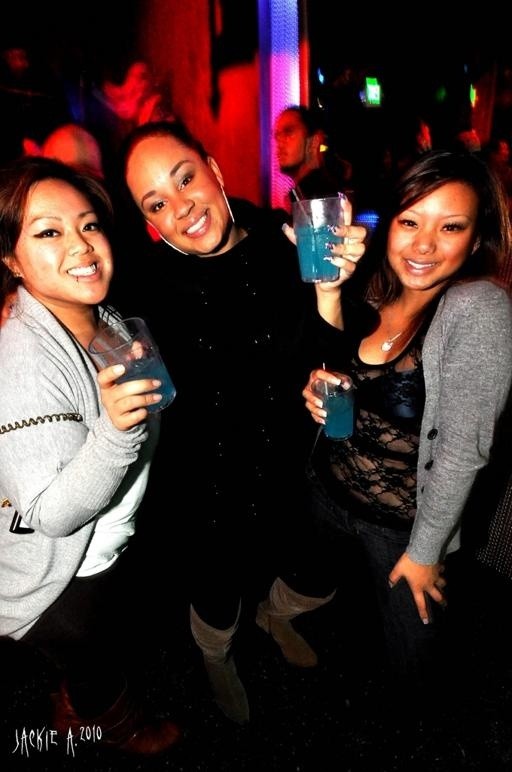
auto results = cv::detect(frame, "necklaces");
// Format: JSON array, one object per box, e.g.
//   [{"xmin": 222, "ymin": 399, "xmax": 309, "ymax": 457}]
[{"xmin": 381, "ymin": 317, "xmax": 402, "ymax": 353}]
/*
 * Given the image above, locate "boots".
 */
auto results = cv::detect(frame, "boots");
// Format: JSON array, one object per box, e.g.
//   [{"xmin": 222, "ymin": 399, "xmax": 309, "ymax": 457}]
[
  {"xmin": 191, "ymin": 597, "xmax": 249, "ymax": 726},
  {"xmin": 254, "ymin": 576, "xmax": 335, "ymax": 668}
]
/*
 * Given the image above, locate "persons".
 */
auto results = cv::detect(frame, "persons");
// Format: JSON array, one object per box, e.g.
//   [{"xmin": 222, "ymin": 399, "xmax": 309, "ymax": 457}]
[
  {"xmin": 0, "ymin": 156, "xmax": 182, "ymax": 755},
  {"xmin": 275, "ymin": 106, "xmax": 348, "ymax": 204},
  {"xmin": 114, "ymin": 120, "xmax": 368, "ymax": 724},
  {"xmin": 1, "ymin": 42, "xmax": 182, "ymax": 174},
  {"xmin": 302, "ymin": 149, "xmax": 512, "ymax": 772},
  {"xmin": 337, "ymin": 121, "xmax": 512, "ymax": 225}
]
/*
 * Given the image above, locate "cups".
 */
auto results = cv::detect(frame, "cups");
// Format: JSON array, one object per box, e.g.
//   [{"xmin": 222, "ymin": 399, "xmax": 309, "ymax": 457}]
[
  {"xmin": 292, "ymin": 197, "xmax": 344, "ymax": 283},
  {"xmin": 87, "ymin": 317, "xmax": 176, "ymax": 414},
  {"xmin": 311, "ymin": 378, "xmax": 355, "ymax": 441}
]
